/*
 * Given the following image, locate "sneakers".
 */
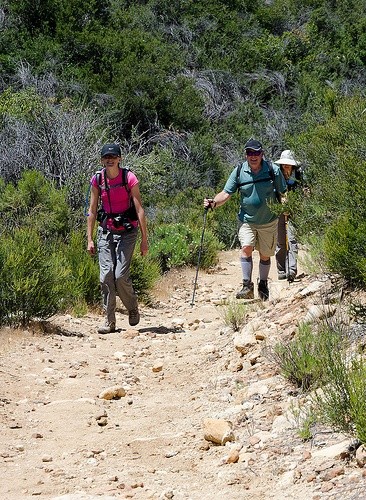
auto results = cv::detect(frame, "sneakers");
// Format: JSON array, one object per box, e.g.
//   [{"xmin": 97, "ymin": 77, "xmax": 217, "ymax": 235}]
[
  {"xmin": 236, "ymin": 279, "xmax": 254, "ymax": 298},
  {"xmin": 257, "ymin": 278, "xmax": 269, "ymax": 301},
  {"xmin": 97, "ymin": 322, "xmax": 115, "ymax": 333},
  {"xmin": 128, "ymin": 305, "xmax": 140, "ymax": 326}
]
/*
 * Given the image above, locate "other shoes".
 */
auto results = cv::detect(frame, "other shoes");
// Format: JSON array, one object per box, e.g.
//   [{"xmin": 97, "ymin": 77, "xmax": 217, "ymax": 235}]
[
  {"xmin": 287, "ymin": 271, "xmax": 295, "ymax": 281},
  {"xmin": 278, "ymin": 271, "xmax": 287, "ymax": 280}
]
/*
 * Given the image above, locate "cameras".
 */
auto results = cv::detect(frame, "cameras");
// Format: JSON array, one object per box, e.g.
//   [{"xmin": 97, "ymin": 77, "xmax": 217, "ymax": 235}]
[{"xmin": 114, "ymin": 216, "xmax": 133, "ymax": 232}]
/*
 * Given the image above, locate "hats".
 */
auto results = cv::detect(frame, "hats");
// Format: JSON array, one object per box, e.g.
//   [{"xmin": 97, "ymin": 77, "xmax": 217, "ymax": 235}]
[
  {"xmin": 273, "ymin": 150, "xmax": 301, "ymax": 167},
  {"xmin": 244, "ymin": 140, "xmax": 262, "ymax": 151},
  {"xmin": 102, "ymin": 144, "xmax": 120, "ymax": 156}
]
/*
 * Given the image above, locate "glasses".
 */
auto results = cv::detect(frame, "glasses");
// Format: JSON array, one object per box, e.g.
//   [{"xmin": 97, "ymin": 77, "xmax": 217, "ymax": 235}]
[
  {"xmin": 246, "ymin": 150, "xmax": 262, "ymax": 156},
  {"xmin": 103, "ymin": 154, "xmax": 117, "ymax": 159}
]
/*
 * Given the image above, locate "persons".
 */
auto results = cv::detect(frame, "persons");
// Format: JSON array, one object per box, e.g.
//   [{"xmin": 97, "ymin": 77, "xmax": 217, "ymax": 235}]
[
  {"xmin": 271, "ymin": 149, "xmax": 306, "ymax": 284},
  {"xmin": 86, "ymin": 144, "xmax": 148, "ymax": 335},
  {"xmin": 203, "ymin": 140, "xmax": 288, "ymax": 303}
]
